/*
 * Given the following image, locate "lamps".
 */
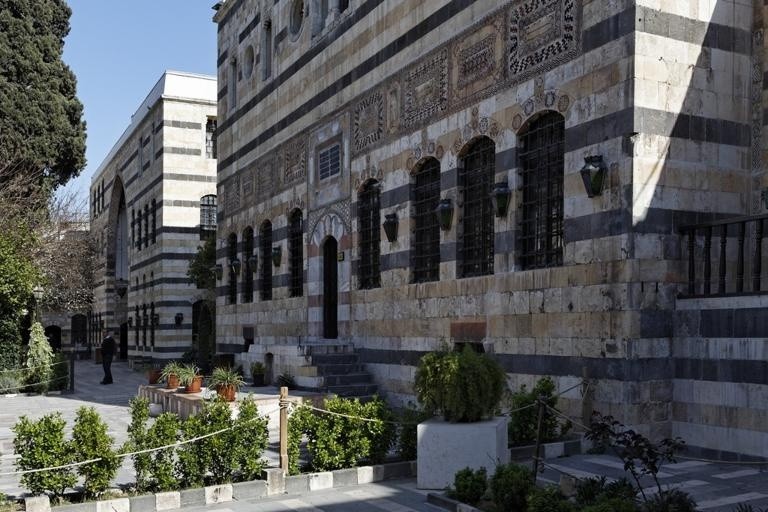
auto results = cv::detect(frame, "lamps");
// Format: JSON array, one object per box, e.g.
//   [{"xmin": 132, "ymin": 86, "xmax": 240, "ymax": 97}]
[
  {"xmin": 382, "ymin": 182, "xmax": 512, "ymax": 240},
  {"xmin": 210, "ymin": 242, "xmax": 282, "ymax": 279},
  {"xmin": 580, "ymin": 154, "xmax": 605, "ymax": 198}
]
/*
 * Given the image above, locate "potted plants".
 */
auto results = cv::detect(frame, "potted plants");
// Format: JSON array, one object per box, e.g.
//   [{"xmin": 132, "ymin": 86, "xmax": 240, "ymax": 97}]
[{"xmin": 156, "ymin": 361, "xmax": 268, "ymax": 404}]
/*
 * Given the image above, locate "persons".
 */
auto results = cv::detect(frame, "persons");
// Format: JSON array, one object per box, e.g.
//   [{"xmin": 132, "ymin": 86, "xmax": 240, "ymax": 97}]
[
  {"xmin": 48, "ymin": 333, "xmax": 55, "ymax": 346},
  {"xmin": 99, "ymin": 328, "xmax": 117, "ymax": 385}
]
